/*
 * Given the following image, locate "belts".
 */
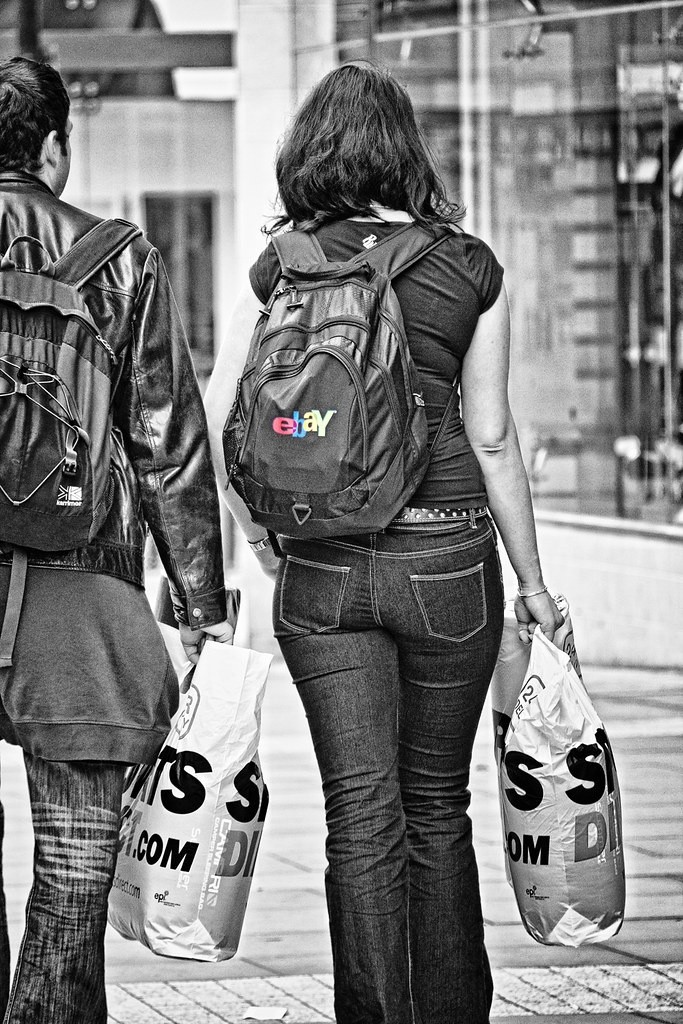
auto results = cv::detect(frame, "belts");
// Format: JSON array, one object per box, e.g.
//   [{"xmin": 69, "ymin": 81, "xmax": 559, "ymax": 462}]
[{"xmin": 391, "ymin": 506, "xmax": 488, "ymax": 524}]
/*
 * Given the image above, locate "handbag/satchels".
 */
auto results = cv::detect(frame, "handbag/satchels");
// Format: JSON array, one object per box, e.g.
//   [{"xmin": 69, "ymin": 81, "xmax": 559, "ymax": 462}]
[
  {"xmin": 491, "ymin": 590, "xmax": 626, "ymax": 948},
  {"xmin": 107, "ymin": 641, "xmax": 273, "ymax": 964}
]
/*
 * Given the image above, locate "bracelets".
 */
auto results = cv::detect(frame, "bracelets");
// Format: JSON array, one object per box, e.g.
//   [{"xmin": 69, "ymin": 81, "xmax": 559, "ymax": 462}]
[
  {"xmin": 247, "ymin": 537, "xmax": 270, "ymax": 552},
  {"xmin": 517, "ymin": 584, "xmax": 547, "ymax": 598}
]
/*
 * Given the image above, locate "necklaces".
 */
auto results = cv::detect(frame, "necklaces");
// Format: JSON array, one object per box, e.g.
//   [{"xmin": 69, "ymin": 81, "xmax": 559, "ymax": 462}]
[{"xmin": 370, "ymin": 205, "xmax": 388, "ymax": 208}]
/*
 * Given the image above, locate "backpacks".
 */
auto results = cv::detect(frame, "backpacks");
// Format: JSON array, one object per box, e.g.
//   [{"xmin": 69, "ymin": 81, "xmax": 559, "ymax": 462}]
[
  {"xmin": 222, "ymin": 221, "xmax": 462, "ymax": 538},
  {"xmin": 0, "ymin": 217, "xmax": 142, "ymax": 551}
]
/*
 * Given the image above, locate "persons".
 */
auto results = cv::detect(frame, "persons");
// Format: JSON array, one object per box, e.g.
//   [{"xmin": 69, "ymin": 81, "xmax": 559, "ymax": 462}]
[
  {"xmin": 0, "ymin": 56, "xmax": 232, "ymax": 1024},
  {"xmin": 204, "ymin": 66, "xmax": 567, "ymax": 1024}
]
